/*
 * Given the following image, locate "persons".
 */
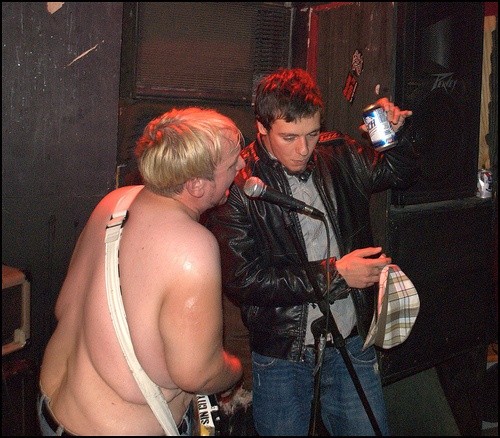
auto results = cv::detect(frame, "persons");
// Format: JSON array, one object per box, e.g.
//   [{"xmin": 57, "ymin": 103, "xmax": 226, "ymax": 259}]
[
  {"xmin": 35, "ymin": 107, "xmax": 244, "ymax": 436},
  {"xmin": 204, "ymin": 67, "xmax": 422, "ymax": 436}
]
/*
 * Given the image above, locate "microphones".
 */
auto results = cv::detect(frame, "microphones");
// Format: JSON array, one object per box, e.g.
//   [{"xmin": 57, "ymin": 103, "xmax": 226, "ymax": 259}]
[{"xmin": 243, "ymin": 177, "xmax": 326, "ymax": 222}]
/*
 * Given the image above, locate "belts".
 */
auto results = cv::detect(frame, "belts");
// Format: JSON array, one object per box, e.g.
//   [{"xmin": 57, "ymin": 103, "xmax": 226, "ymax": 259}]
[
  {"xmin": 302, "ymin": 323, "xmax": 359, "ymax": 350},
  {"xmin": 35, "ymin": 379, "xmax": 77, "ymax": 437}
]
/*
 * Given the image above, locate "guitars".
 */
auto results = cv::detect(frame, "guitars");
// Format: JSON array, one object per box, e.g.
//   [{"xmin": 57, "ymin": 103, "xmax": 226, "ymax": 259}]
[{"xmin": 192, "ymin": 394, "xmax": 221, "ymax": 436}]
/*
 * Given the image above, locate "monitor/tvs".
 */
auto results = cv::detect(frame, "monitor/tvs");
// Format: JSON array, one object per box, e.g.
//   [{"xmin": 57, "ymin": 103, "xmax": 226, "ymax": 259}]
[
  {"xmin": 1, "ymin": 263, "xmax": 31, "ymax": 356},
  {"xmin": 378, "ymin": 194, "xmax": 500, "ymax": 385}
]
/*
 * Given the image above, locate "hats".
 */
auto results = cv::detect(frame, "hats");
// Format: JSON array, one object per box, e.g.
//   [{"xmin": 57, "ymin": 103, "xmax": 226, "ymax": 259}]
[{"xmin": 360, "ymin": 263, "xmax": 421, "ymax": 350}]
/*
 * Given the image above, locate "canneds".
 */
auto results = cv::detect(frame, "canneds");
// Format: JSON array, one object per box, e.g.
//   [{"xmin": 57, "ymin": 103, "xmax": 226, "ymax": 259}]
[
  {"xmin": 476, "ymin": 169, "xmax": 493, "ymax": 198},
  {"xmin": 361, "ymin": 104, "xmax": 398, "ymax": 152}
]
